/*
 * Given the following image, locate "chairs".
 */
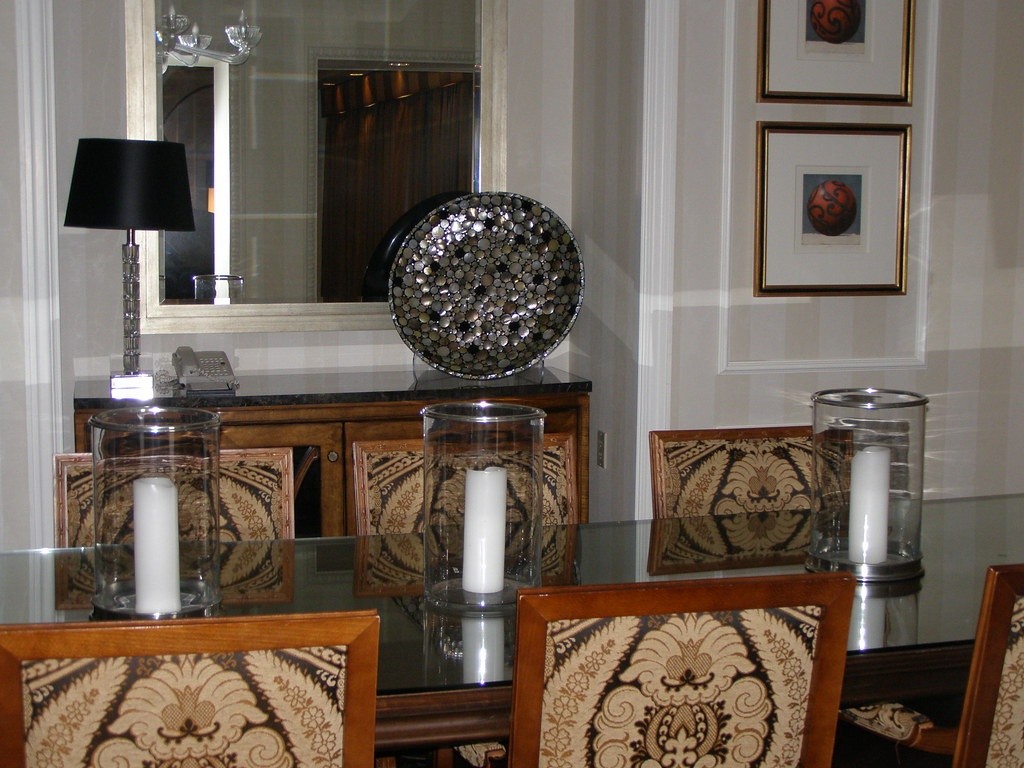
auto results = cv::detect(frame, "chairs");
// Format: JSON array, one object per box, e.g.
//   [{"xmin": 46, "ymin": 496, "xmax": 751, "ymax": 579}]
[
  {"xmin": 434, "ymin": 571, "xmax": 855, "ymax": 768},
  {"xmin": 648, "ymin": 427, "xmax": 815, "ymax": 518},
  {"xmin": 830, "ymin": 562, "xmax": 1024, "ymax": 768},
  {"xmin": 347, "ymin": 433, "xmax": 579, "ymax": 527},
  {"xmin": 54, "ymin": 445, "xmax": 297, "ymax": 545},
  {"xmin": 0, "ymin": 609, "xmax": 382, "ymax": 768}
]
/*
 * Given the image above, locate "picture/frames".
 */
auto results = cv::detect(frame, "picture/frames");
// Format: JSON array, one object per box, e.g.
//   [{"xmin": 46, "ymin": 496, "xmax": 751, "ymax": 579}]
[
  {"xmin": 753, "ymin": 121, "xmax": 911, "ymax": 296},
  {"xmin": 755, "ymin": 0, "xmax": 913, "ymax": 108}
]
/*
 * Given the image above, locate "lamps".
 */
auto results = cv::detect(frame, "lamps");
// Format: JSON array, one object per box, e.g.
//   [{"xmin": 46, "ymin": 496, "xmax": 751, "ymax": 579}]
[
  {"xmin": 61, "ymin": 138, "xmax": 196, "ymax": 388},
  {"xmin": 358, "ymin": 72, "xmax": 378, "ymax": 109},
  {"xmin": 331, "ymin": 85, "xmax": 351, "ymax": 117},
  {"xmin": 389, "ymin": 71, "xmax": 415, "ymax": 100},
  {"xmin": 156, "ymin": 3, "xmax": 262, "ymax": 75}
]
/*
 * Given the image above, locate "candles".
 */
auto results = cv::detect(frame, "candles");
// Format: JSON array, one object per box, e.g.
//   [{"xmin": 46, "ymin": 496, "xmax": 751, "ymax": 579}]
[
  {"xmin": 88, "ymin": 407, "xmax": 222, "ymax": 621},
  {"xmin": 421, "ymin": 400, "xmax": 548, "ymax": 613},
  {"xmin": 803, "ymin": 386, "xmax": 929, "ymax": 583},
  {"xmin": 446, "ymin": 608, "xmax": 518, "ymax": 685},
  {"xmin": 847, "ymin": 578, "xmax": 920, "ymax": 652}
]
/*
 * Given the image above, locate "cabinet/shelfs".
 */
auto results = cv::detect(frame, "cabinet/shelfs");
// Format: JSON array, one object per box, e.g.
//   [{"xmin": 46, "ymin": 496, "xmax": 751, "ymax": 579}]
[{"xmin": 74, "ymin": 393, "xmax": 591, "ymax": 537}]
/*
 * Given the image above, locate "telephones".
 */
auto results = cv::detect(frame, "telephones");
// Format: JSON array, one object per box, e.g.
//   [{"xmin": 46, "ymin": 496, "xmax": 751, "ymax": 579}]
[{"xmin": 171, "ymin": 346, "xmax": 240, "ymax": 391}]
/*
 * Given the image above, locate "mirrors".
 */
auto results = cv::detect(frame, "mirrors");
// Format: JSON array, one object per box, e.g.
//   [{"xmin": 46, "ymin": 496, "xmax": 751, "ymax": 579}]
[{"xmin": 126, "ymin": 0, "xmax": 505, "ymax": 337}]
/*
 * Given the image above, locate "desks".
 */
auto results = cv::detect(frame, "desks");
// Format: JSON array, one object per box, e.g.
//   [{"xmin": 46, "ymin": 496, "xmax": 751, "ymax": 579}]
[{"xmin": 0, "ymin": 491, "xmax": 1024, "ymax": 747}]
